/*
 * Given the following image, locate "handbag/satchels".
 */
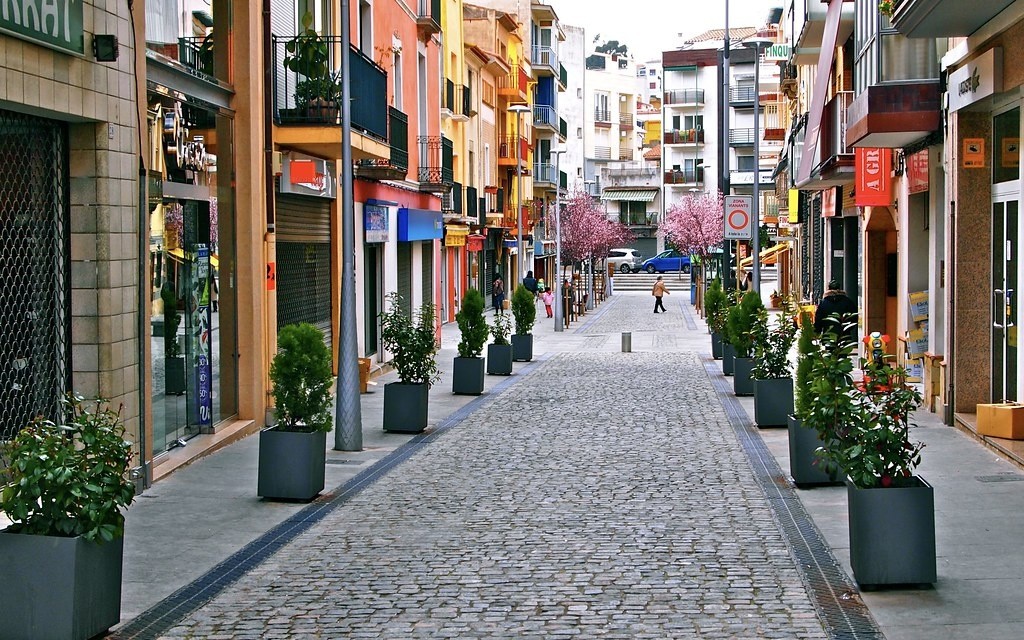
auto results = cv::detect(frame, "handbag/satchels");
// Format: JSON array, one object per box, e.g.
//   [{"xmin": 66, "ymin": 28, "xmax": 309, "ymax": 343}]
[{"xmin": 740, "ymin": 277, "xmax": 748, "ymax": 291}]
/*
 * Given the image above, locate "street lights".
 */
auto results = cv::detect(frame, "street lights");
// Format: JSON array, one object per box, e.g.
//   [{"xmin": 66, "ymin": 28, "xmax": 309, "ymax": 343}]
[
  {"xmin": 549, "ymin": 148, "xmax": 568, "ymax": 334},
  {"xmin": 506, "ymin": 105, "xmax": 532, "ymax": 289},
  {"xmin": 742, "ymin": 36, "xmax": 774, "ymax": 300}
]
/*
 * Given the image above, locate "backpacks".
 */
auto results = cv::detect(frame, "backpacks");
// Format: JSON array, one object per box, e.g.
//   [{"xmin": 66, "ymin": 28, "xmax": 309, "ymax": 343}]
[{"xmin": 493, "ymin": 282, "xmax": 503, "ymax": 297}]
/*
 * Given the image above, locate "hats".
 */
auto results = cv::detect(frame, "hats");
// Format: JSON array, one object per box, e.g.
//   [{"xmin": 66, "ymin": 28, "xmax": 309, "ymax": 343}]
[{"xmin": 828, "ymin": 278, "xmax": 841, "ymax": 290}]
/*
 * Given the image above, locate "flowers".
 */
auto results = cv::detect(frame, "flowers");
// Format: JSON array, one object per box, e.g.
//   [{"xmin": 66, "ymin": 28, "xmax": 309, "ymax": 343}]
[{"xmin": 877, "ymin": 0, "xmax": 895, "ymax": 17}]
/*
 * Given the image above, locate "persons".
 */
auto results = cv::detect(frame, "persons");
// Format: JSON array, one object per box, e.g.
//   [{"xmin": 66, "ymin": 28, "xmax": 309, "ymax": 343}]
[
  {"xmin": 543, "ymin": 287, "xmax": 553, "ymax": 318},
  {"xmin": 492, "ymin": 273, "xmax": 504, "ymax": 315},
  {"xmin": 813, "ymin": 280, "xmax": 855, "ymax": 357},
  {"xmin": 561, "ymin": 280, "xmax": 574, "ymax": 325},
  {"xmin": 523, "ymin": 271, "xmax": 538, "ymax": 294},
  {"xmin": 652, "ymin": 276, "xmax": 670, "ymax": 313},
  {"xmin": 211, "ymin": 274, "xmax": 218, "ymax": 312},
  {"xmin": 730, "ymin": 270, "xmax": 752, "ymax": 291}
]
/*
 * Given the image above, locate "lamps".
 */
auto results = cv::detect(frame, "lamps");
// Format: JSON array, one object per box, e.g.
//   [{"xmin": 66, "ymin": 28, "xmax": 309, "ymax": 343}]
[{"xmin": 92, "ymin": 33, "xmax": 121, "ymax": 62}]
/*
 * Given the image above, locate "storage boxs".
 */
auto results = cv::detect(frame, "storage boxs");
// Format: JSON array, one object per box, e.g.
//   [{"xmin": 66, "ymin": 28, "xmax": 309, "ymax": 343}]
[
  {"xmin": 358, "ymin": 357, "xmax": 371, "ymax": 393},
  {"xmin": 770, "ymin": 297, "xmax": 782, "ymax": 308},
  {"xmin": 905, "ymin": 290, "xmax": 928, "ymax": 383},
  {"xmin": 976, "ymin": 402, "xmax": 1024, "ymax": 441}
]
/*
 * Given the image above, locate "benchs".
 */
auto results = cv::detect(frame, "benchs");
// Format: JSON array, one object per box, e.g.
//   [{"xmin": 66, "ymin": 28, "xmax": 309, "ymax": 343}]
[{"xmin": 575, "ymin": 294, "xmax": 588, "ymax": 313}]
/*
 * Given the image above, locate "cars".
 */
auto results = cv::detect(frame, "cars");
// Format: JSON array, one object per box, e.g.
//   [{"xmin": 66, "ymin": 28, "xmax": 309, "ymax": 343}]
[{"xmin": 641, "ymin": 249, "xmax": 693, "ymax": 274}]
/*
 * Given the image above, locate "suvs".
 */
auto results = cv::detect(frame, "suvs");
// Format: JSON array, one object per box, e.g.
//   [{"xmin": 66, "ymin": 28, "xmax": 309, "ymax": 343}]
[{"xmin": 581, "ymin": 248, "xmax": 643, "ymax": 274}]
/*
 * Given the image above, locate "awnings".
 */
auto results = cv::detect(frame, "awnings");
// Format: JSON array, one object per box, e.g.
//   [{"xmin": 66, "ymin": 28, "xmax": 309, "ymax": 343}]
[{"xmin": 740, "ymin": 243, "xmax": 787, "ymax": 268}]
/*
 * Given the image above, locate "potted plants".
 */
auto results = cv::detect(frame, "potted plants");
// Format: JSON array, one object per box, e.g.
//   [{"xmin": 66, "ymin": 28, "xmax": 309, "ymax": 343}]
[
  {"xmin": 484, "ymin": 185, "xmax": 498, "ymax": 194},
  {"xmin": 451, "ymin": 287, "xmax": 490, "ymax": 396},
  {"xmin": 810, "ymin": 312, "xmax": 937, "ymax": 586},
  {"xmin": 283, "ymin": 12, "xmax": 343, "ymax": 124},
  {"xmin": 0, "ymin": 390, "xmax": 145, "ymax": 640},
  {"xmin": 486, "ymin": 284, "xmax": 536, "ymax": 375},
  {"xmin": 506, "ymin": 201, "xmax": 533, "ymax": 229},
  {"xmin": 257, "ymin": 321, "xmax": 334, "ymax": 503},
  {"xmin": 376, "ymin": 292, "xmax": 444, "ymax": 432},
  {"xmin": 786, "ymin": 309, "xmax": 848, "ymax": 486},
  {"xmin": 706, "ymin": 274, "xmax": 799, "ymax": 428}
]
